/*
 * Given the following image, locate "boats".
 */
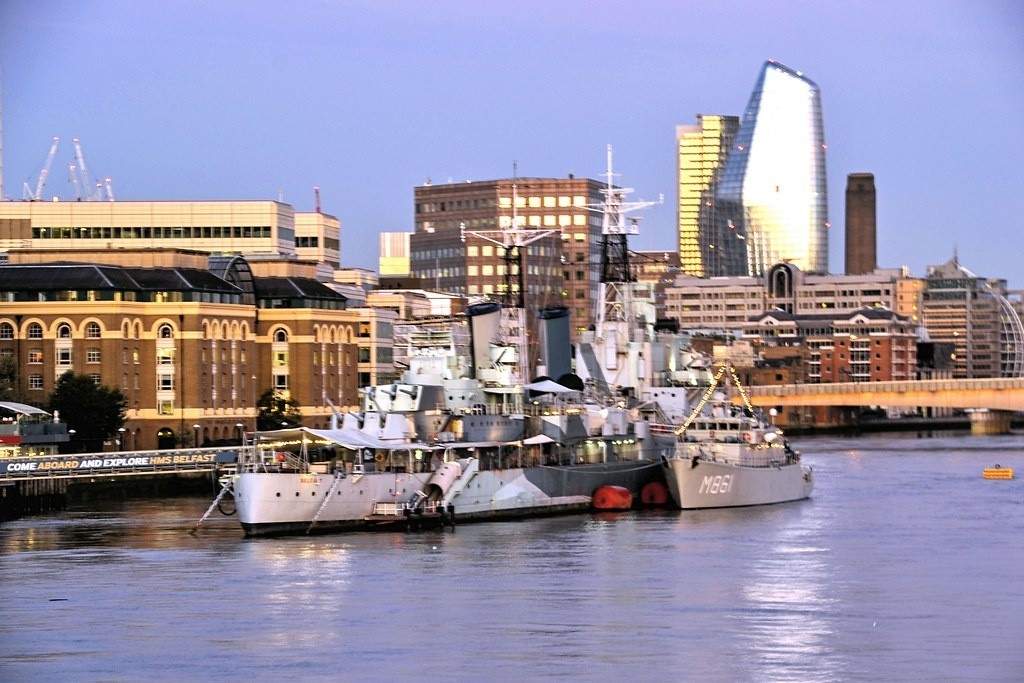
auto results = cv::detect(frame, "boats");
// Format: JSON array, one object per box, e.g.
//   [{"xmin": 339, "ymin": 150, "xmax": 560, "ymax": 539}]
[
  {"xmin": 659, "ymin": 327, "xmax": 811, "ymax": 509},
  {"xmin": 229, "ymin": 143, "xmax": 786, "ymax": 539}
]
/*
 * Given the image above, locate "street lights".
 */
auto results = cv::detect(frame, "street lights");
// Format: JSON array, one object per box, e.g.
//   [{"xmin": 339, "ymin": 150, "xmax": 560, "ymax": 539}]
[
  {"xmin": 234, "ymin": 423, "xmax": 244, "ymax": 445},
  {"xmin": 118, "ymin": 427, "xmax": 126, "ymax": 452},
  {"xmin": 280, "ymin": 421, "xmax": 289, "ymax": 430},
  {"xmin": 192, "ymin": 424, "xmax": 200, "ymax": 448},
  {"xmin": 69, "ymin": 430, "xmax": 77, "ymax": 455}
]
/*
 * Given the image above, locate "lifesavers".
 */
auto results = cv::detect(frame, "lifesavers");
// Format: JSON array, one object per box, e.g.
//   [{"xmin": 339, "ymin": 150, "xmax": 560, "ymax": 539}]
[
  {"xmin": 277, "ymin": 453, "xmax": 286, "ymax": 461},
  {"xmin": 374, "ymin": 453, "xmax": 385, "ymax": 463},
  {"xmin": 742, "ymin": 433, "xmax": 752, "ymax": 442}
]
